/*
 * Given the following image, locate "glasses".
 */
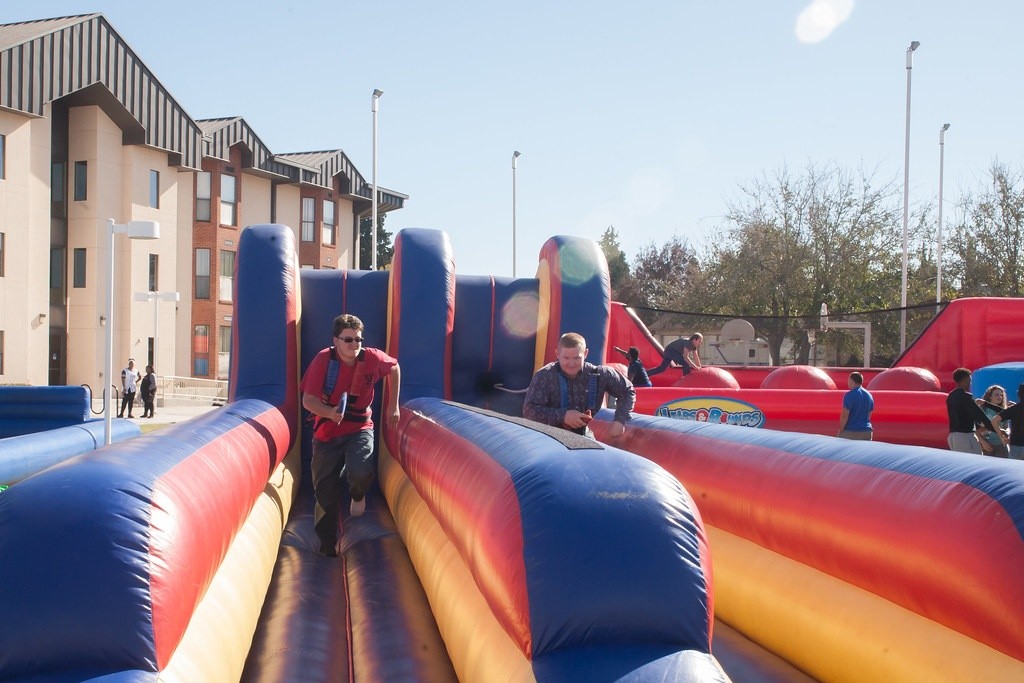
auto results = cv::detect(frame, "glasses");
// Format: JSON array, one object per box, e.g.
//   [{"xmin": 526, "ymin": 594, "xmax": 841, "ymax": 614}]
[{"xmin": 337, "ymin": 336, "xmax": 365, "ymax": 343}]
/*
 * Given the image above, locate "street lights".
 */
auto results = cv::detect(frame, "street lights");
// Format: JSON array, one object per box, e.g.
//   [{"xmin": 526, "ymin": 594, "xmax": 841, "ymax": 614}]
[
  {"xmin": 133, "ymin": 291, "xmax": 181, "ymax": 376},
  {"xmin": 103, "ymin": 218, "xmax": 161, "ymax": 444},
  {"xmin": 512, "ymin": 150, "xmax": 522, "ymax": 278},
  {"xmin": 370, "ymin": 87, "xmax": 384, "ymax": 271},
  {"xmin": 936, "ymin": 123, "xmax": 952, "ymax": 315},
  {"xmin": 898, "ymin": 41, "xmax": 923, "ymax": 352}
]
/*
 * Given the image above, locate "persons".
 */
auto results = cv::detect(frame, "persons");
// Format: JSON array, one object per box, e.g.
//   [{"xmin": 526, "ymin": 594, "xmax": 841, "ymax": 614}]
[
  {"xmin": 299, "ymin": 314, "xmax": 401, "ymax": 557},
  {"xmin": 992, "ymin": 384, "xmax": 1024, "ymax": 460},
  {"xmin": 613, "ymin": 345, "xmax": 652, "ymax": 388},
  {"xmin": 946, "ymin": 368, "xmax": 1008, "ymax": 455},
  {"xmin": 975, "ymin": 385, "xmax": 1011, "ymax": 459},
  {"xmin": 117, "ymin": 359, "xmax": 141, "ymax": 418},
  {"xmin": 647, "ymin": 333, "xmax": 704, "ymax": 377},
  {"xmin": 523, "ymin": 333, "xmax": 636, "ymax": 442},
  {"xmin": 141, "ymin": 365, "xmax": 157, "ymax": 418},
  {"xmin": 837, "ymin": 372, "xmax": 874, "ymax": 441}
]
